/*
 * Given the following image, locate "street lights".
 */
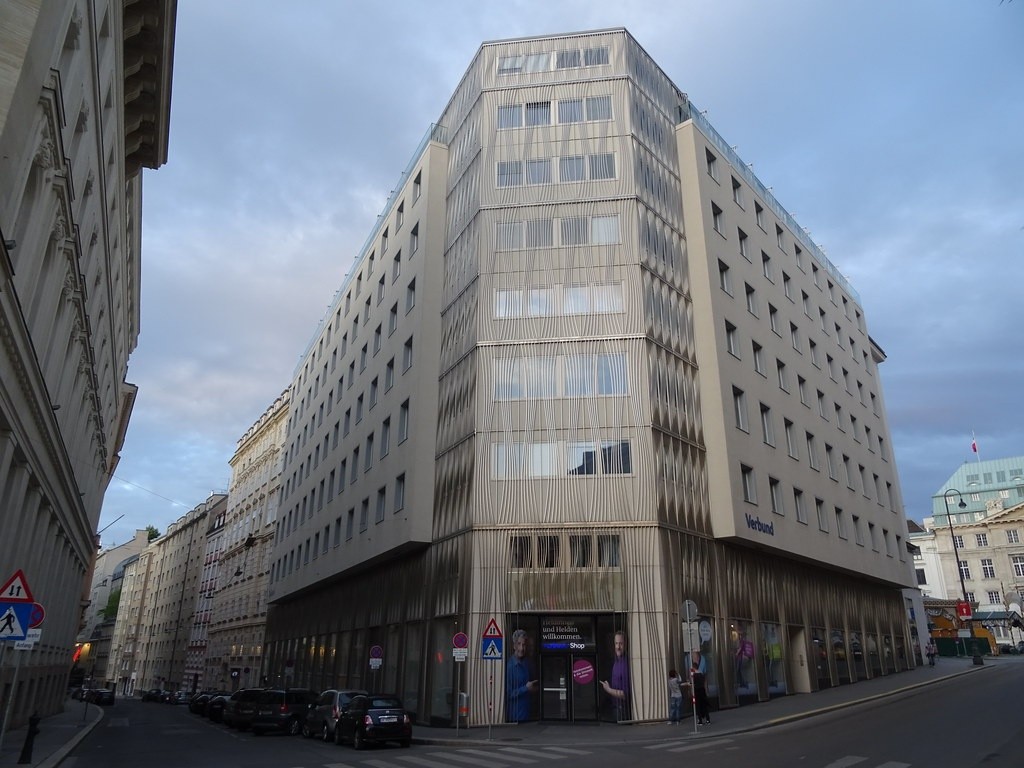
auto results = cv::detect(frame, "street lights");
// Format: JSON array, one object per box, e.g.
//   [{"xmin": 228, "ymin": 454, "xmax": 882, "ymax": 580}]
[{"xmin": 944, "ymin": 489, "xmax": 985, "ymax": 665}]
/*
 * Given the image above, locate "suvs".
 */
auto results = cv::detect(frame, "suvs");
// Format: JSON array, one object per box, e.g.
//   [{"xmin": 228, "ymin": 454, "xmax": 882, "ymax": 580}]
[
  {"xmin": 233, "ymin": 687, "xmax": 266, "ymax": 732},
  {"xmin": 249, "ymin": 687, "xmax": 320, "ymax": 737},
  {"xmin": 223, "ymin": 688, "xmax": 244, "ymax": 729},
  {"xmin": 301, "ymin": 688, "xmax": 368, "ymax": 743}
]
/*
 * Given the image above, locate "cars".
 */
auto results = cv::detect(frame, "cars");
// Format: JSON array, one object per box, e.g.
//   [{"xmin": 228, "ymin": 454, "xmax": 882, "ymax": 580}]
[
  {"xmin": 819, "ymin": 641, "xmax": 905, "ymax": 660},
  {"xmin": 334, "ymin": 694, "xmax": 413, "ymax": 750},
  {"xmin": 71, "ymin": 684, "xmax": 115, "ymax": 706},
  {"xmin": 188, "ymin": 689, "xmax": 233, "ymax": 723},
  {"xmin": 143, "ymin": 688, "xmax": 192, "ymax": 706}
]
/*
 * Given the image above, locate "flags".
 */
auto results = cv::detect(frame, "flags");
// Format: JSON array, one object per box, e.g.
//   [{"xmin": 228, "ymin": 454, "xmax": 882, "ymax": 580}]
[{"xmin": 972, "ymin": 442, "xmax": 977, "ymax": 453}]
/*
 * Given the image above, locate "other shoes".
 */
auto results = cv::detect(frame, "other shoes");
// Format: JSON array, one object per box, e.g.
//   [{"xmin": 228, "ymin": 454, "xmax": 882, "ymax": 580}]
[
  {"xmin": 705, "ymin": 721, "xmax": 710, "ymax": 725},
  {"xmin": 667, "ymin": 721, "xmax": 672, "ymax": 725},
  {"xmin": 677, "ymin": 722, "xmax": 682, "ymax": 726},
  {"xmin": 697, "ymin": 722, "xmax": 702, "ymax": 726}
]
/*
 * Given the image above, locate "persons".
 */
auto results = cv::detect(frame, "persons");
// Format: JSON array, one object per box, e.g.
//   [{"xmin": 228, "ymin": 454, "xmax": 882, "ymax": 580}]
[
  {"xmin": 689, "ymin": 652, "xmax": 710, "ymax": 726},
  {"xmin": 914, "ymin": 645, "xmax": 922, "ymax": 666},
  {"xmin": 925, "ymin": 641, "xmax": 939, "ymax": 671},
  {"xmin": 507, "ymin": 629, "xmax": 539, "ymax": 720},
  {"xmin": 667, "ymin": 671, "xmax": 682, "ymax": 726},
  {"xmin": 600, "ymin": 631, "xmax": 630, "ymax": 721}
]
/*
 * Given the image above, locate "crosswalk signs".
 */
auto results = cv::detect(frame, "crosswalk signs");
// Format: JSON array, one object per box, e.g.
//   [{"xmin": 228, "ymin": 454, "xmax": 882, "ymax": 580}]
[
  {"xmin": 0, "ymin": 599, "xmax": 34, "ymax": 641},
  {"xmin": 482, "ymin": 638, "xmax": 503, "ymax": 659}
]
